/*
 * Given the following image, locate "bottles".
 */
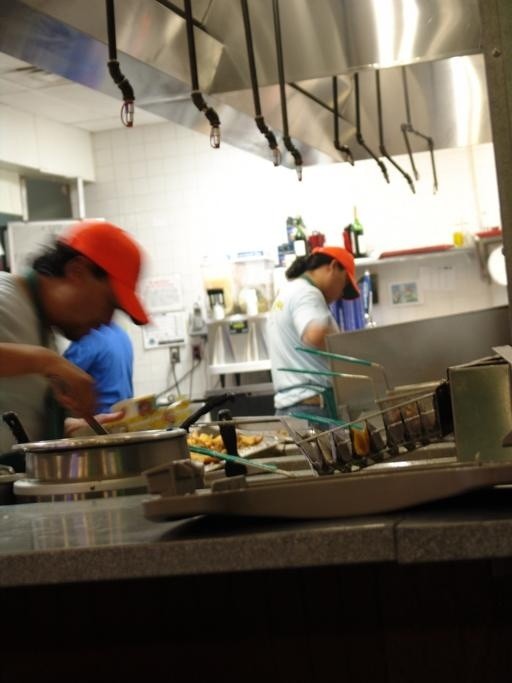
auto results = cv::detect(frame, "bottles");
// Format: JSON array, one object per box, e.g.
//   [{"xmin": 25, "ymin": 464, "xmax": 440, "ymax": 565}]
[
  {"xmin": 293, "ymin": 215, "xmax": 309, "ymax": 261},
  {"xmin": 350, "ymin": 204, "xmax": 367, "ymax": 256}
]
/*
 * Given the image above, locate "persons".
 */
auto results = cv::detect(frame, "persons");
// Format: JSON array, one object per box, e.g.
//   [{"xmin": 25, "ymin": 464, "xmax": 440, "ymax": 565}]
[
  {"xmin": 265, "ymin": 246, "xmax": 360, "ymax": 433},
  {"xmin": 0, "ymin": 217, "xmax": 150, "ymax": 506},
  {"xmin": 54, "ymin": 318, "xmax": 134, "ymax": 435}
]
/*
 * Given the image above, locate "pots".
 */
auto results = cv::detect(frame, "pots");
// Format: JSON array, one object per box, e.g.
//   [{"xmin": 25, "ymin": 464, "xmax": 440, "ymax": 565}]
[{"xmin": 10, "ymin": 431, "xmax": 194, "ymax": 499}]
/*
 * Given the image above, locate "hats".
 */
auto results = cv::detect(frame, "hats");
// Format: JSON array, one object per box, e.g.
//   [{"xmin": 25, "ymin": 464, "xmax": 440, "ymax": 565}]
[
  {"xmin": 56, "ymin": 221, "xmax": 147, "ymax": 328},
  {"xmin": 311, "ymin": 246, "xmax": 360, "ymax": 299}
]
